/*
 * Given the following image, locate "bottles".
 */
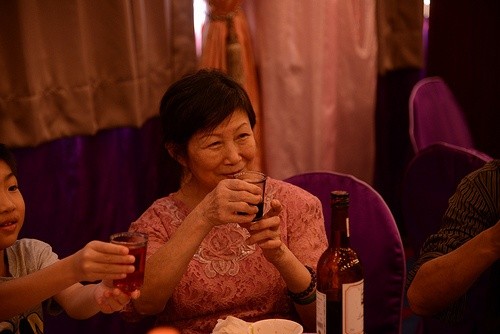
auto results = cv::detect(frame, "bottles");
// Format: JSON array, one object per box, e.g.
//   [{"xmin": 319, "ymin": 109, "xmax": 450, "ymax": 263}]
[{"xmin": 316, "ymin": 190, "xmax": 367, "ymax": 334}]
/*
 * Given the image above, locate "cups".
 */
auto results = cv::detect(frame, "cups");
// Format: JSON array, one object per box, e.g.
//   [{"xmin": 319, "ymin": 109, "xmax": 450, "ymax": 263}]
[
  {"xmin": 233, "ymin": 171, "xmax": 266, "ymax": 222},
  {"xmin": 248, "ymin": 319, "xmax": 303, "ymax": 334},
  {"xmin": 109, "ymin": 230, "xmax": 151, "ymax": 294}
]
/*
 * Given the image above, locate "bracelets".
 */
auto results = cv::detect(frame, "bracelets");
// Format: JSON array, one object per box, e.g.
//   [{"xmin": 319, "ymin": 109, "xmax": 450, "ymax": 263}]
[{"xmin": 285, "ymin": 265, "xmax": 319, "ymax": 299}]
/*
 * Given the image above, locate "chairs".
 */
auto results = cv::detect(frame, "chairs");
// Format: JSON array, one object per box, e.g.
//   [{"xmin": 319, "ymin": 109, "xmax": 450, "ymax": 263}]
[
  {"xmin": 408, "ymin": 76, "xmax": 474, "ymax": 154},
  {"xmin": 402, "ymin": 142, "xmax": 495, "ymax": 266},
  {"xmin": 280, "ymin": 171, "xmax": 407, "ymax": 334}
]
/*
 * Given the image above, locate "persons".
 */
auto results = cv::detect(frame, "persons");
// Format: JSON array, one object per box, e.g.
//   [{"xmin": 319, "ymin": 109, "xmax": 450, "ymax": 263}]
[
  {"xmin": 403, "ymin": 158, "xmax": 499, "ymax": 334},
  {"xmin": 0, "ymin": 156, "xmax": 141, "ymax": 334},
  {"xmin": 128, "ymin": 68, "xmax": 330, "ymax": 334}
]
